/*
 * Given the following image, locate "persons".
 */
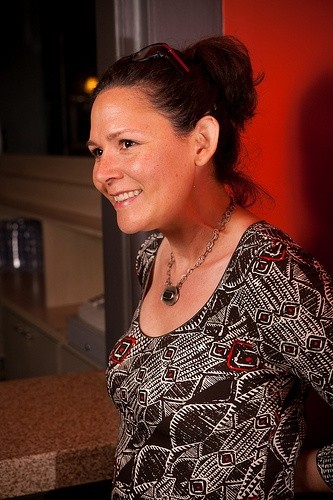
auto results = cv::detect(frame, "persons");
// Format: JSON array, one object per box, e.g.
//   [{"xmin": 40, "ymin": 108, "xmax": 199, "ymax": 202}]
[{"xmin": 87, "ymin": 35, "xmax": 333, "ymax": 500}]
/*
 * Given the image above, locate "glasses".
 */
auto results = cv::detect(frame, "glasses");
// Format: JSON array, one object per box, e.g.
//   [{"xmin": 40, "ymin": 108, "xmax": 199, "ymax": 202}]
[{"xmin": 134, "ymin": 42, "xmax": 219, "ymax": 111}]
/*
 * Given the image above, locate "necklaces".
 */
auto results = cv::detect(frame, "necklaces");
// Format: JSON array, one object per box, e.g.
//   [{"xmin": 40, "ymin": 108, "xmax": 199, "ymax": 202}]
[{"xmin": 161, "ymin": 200, "xmax": 235, "ymax": 306}]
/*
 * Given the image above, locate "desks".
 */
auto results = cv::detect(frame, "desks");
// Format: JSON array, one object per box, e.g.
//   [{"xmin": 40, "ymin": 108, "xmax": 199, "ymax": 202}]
[{"xmin": 0, "ymin": 370, "xmax": 122, "ymax": 500}]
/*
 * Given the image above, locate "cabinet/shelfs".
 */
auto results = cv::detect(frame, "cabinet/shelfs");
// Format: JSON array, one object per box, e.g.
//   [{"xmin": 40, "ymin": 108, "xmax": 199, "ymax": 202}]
[{"xmin": 1, "ymin": 0, "xmax": 224, "ymax": 376}]
